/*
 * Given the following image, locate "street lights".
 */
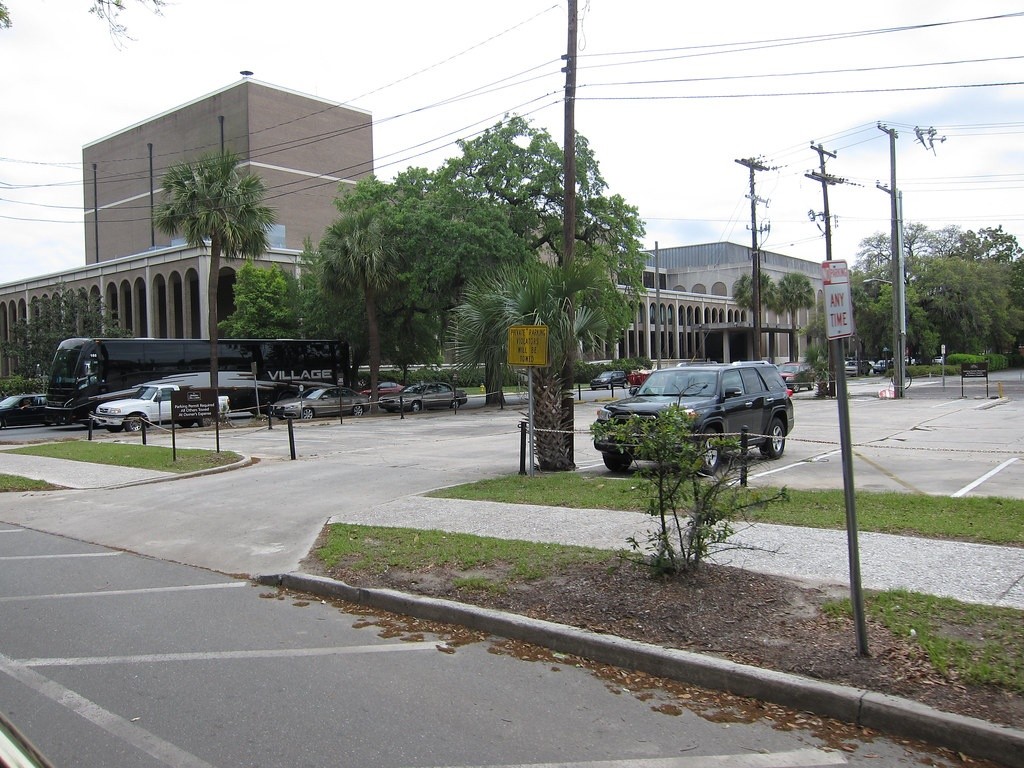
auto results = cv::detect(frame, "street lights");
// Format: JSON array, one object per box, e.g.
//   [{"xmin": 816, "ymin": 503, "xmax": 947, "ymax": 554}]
[{"xmin": 862, "ymin": 278, "xmax": 907, "ymax": 399}]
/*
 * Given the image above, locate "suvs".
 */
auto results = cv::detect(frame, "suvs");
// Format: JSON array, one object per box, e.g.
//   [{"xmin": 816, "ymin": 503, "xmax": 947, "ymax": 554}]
[
  {"xmin": 591, "ymin": 360, "xmax": 796, "ymax": 477},
  {"xmin": 589, "ymin": 370, "xmax": 630, "ymax": 392},
  {"xmin": 776, "ymin": 361, "xmax": 818, "ymax": 394}
]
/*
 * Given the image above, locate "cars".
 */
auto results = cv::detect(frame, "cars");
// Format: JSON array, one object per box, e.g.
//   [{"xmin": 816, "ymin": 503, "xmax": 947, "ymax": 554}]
[
  {"xmin": 845, "ymin": 360, "xmax": 872, "ymax": 378},
  {"xmin": 0, "ymin": 393, "xmax": 62, "ymax": 428},
  {"xmin": 271, "ymin": 385, "xmax": 371, "ymax": 420},
  {"xmin": 378, "ymin": 381, "xmax": 469, "ymax": 414},
  {"xmin": 354, "ymin": 380, "xmax": 406, "ymax": 400},
  {"xmin": 932, "ymin": 356, "xmax": 944, "ymax": 365},
  {"xmin": 872, "ymin": 359, "xmax": 895, "ymax": 374}
]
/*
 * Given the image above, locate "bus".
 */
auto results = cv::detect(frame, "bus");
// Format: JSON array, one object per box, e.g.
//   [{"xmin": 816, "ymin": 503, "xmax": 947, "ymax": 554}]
[{"xmin": 43, "ymin": 336, "xmax": 354, "ymax": 425}]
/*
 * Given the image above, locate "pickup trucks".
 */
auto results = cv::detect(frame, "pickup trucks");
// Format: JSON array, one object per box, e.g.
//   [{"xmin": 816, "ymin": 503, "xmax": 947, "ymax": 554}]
[{"xmin": 94, "ymin": 383, "xmax": 232, "ymax": 432}]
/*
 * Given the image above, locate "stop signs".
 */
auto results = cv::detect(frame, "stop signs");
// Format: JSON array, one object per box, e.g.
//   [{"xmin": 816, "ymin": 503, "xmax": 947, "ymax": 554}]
[{"xmin": 878, "ymin": 389, "xmax": 895, "ymax": 400}]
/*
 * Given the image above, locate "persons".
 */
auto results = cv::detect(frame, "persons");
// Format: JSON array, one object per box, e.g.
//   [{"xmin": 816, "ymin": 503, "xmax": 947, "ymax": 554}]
[{"xmin": 20, "ymin": 399, "xmax": 31, "ymax": 410}]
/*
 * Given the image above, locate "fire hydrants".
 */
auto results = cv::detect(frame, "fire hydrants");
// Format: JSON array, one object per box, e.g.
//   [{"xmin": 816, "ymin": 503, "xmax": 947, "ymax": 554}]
[{"xmin": 479, "ymin": 383, "xmax": 485, "ymax": 392}]
